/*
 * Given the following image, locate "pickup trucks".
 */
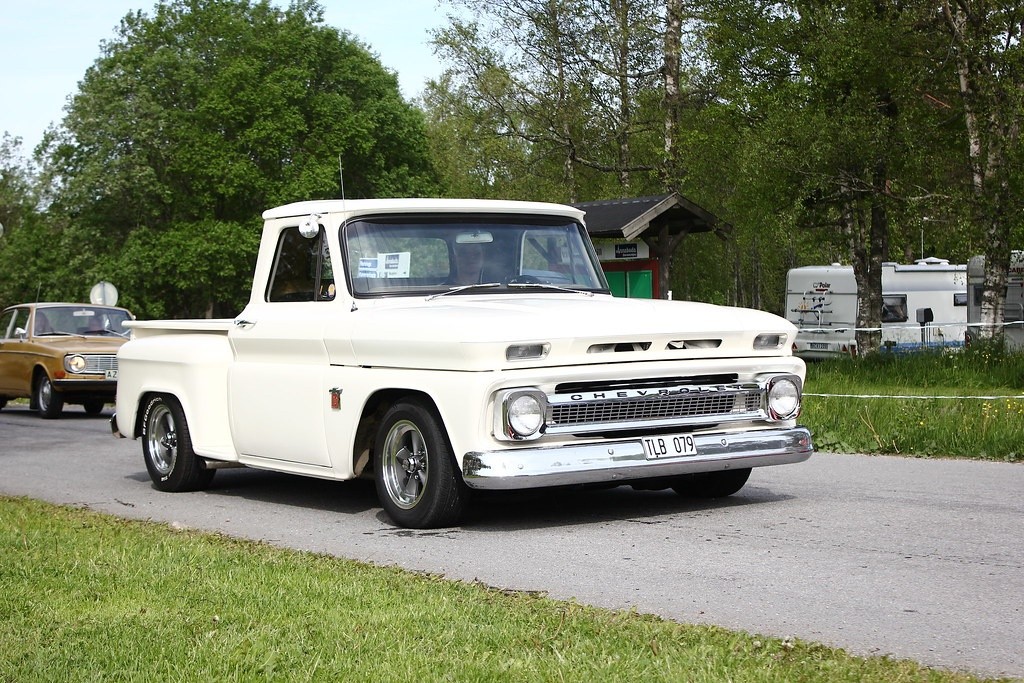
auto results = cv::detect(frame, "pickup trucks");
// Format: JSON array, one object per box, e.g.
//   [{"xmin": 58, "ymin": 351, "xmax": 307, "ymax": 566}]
[{"xmin": 108, "ymin": 196, "xmax": 813, "ymax": 530}]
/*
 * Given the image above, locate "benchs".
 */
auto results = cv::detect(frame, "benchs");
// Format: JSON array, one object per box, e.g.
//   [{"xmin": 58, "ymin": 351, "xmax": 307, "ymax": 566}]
[{"xmin": 266, "ymin": 275, "xmax": 449, "ymax": 298}]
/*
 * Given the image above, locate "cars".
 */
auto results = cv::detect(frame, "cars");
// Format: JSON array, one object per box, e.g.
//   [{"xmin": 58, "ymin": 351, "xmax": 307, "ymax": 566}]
[{"xmin": 0, "ymin": 303, "xmax": 136, "ymax": 415}]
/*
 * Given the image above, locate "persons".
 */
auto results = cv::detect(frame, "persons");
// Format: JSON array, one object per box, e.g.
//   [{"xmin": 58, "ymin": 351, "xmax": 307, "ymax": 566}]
[
  {"xmin": 440, "ymin": 237, "xmax": 483, "ymax": 285},
  {"xmin": 35, "ymin": 312, "xmax": 47, "ymax": 334},
  {"xmin": 84, "ymin": 316, "xmax": 102, "ymax": 332}
]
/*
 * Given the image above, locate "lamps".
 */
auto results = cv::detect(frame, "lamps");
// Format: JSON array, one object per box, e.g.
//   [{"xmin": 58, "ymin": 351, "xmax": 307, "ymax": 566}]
[{"xmin": 916, "ymin": 307, "xmax": 934, "ymax": 349}]
